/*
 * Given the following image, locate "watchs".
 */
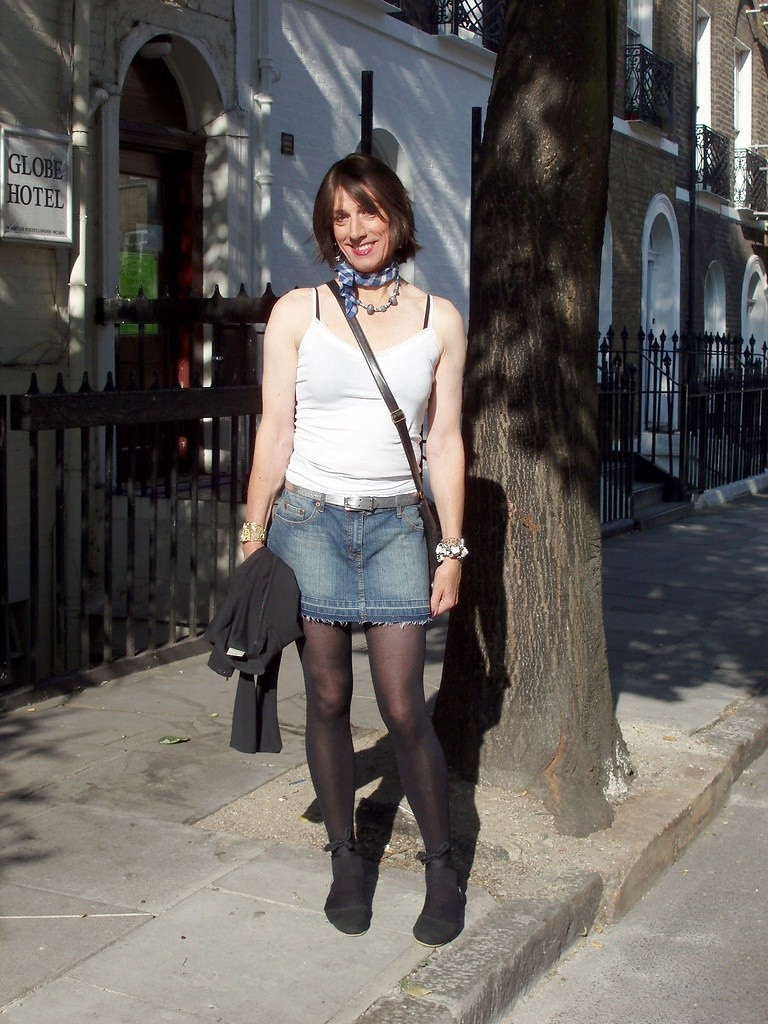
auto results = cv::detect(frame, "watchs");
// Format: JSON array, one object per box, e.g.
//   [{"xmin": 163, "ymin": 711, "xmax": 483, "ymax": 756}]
[{"xmin": 435, "ymin": 537, "xmax": 470, "ymax": 563}]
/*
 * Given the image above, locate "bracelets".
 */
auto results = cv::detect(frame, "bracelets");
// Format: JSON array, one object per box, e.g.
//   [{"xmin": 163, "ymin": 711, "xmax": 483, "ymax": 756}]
[{"xmin": 240, "ymin": 521, "xmax": 268, "ymax": 543}]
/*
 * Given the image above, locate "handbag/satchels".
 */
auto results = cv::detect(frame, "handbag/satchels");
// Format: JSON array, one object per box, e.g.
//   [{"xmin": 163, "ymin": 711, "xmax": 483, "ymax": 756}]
[{"xmin": 418, "ymin": 496, "xmax": 443, "ymax": 597}]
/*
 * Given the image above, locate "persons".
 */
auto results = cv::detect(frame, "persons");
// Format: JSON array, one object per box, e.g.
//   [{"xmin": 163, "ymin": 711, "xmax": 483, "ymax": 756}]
[{"xmin": 237, "ymin": 150, "xmax": 471, "ymax": 950}]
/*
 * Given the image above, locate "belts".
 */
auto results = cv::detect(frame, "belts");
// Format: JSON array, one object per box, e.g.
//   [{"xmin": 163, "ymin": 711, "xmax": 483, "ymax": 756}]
[{"xmin": 285, "ymin": 480, "xmax": 421, "ymax": 512}]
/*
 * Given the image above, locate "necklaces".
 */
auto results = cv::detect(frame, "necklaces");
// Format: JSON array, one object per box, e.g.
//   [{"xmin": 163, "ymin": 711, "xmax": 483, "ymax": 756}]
[{"xmin": 355, "ymin": 274, "xmax": 401, "ymax": 315}]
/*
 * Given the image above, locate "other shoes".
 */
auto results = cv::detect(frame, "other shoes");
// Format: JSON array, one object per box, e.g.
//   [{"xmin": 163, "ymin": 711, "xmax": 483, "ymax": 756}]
[
  {"xmin": 323, "ymin": 828, "xmax": 370, "ymax": 937},
  {"xmin": 413, "ymin": 843, "xmax": 461, "ymax": 948}
]
